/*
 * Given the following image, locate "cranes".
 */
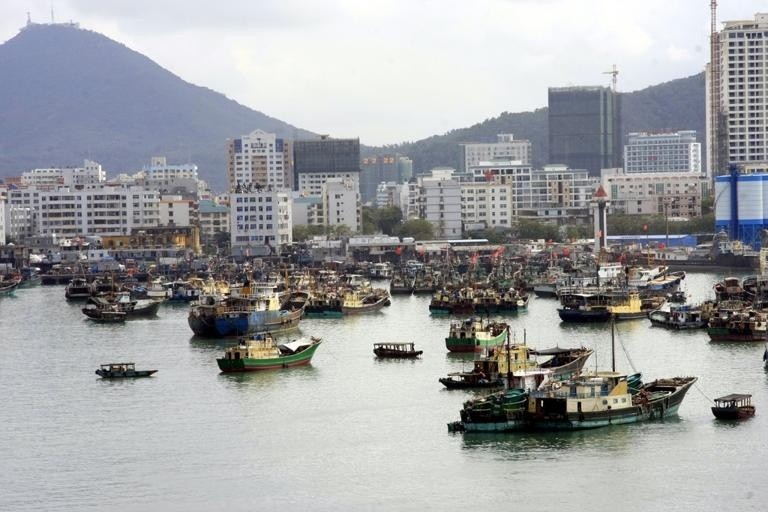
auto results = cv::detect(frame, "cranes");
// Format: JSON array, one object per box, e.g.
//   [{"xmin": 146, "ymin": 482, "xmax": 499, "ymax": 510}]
[
  {"xmin": 602, "ymin": 64, "xmax": 619, "ymax": 167},
  {"xmin": 708, "ymin": 0, "xmax": 719, "ymax": 176}
]
